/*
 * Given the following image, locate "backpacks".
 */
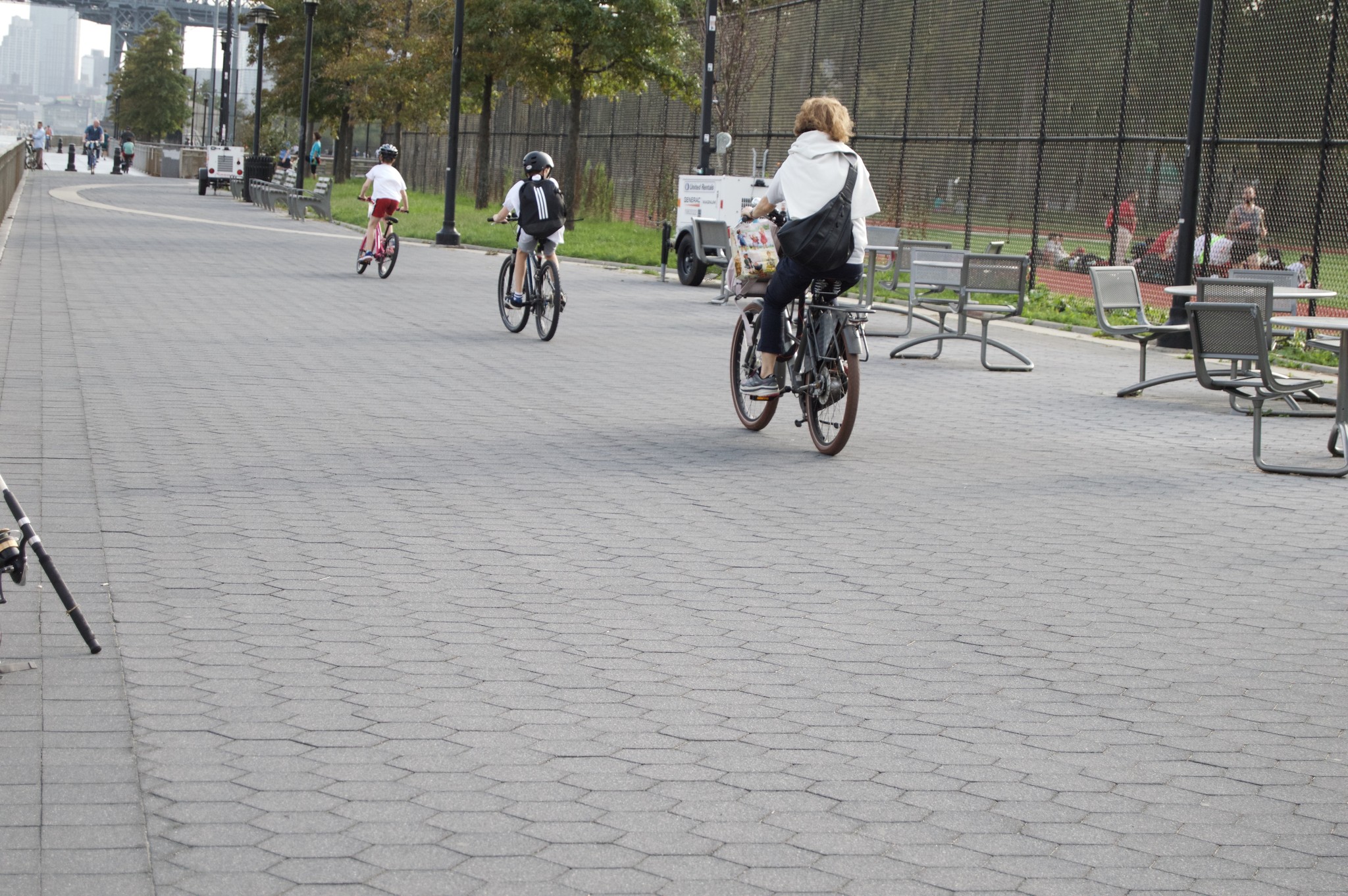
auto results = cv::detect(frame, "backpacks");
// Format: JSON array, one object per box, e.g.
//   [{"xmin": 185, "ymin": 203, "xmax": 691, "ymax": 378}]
[
  {"xmin": 518, "ymin": 178, "xmax": 566, "ymax": 238},
  {"xmin": 725, "ymin": 216, "xmax": 781, "ymax": 297}
]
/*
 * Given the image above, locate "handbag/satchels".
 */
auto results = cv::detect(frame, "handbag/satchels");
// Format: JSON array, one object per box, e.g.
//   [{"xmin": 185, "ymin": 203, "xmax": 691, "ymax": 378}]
[
  {"xmin": 316, "ymin": 157, "xmax": 320, "ymax": 164},
  {"xmin": 729, "ymin": 218, "xmax": 779, "ymax": 281},
  {"xmin": 777, "ymin": 195, "xmax": 855, "ymax": 273}
]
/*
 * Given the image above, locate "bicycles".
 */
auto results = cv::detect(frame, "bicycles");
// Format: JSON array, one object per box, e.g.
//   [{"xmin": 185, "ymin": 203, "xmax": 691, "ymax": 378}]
[
  {"xmin": 486, "ymin": 212, "xmax": 567, "ymax": 341},
  {"xmin": 46, "ymin": 135, "xmax": 51, "ymax": 151},
  {"xmin": 118, "ymin": 143, "xmax": 134, "ymax": 173},
  {"xmin": 25, "ymin": 137, "xmax": 38, "ymax": 169},
  {"xmin": 728, "ymin": 209, "xmax": 875, "ymax": 455},
  {"xmin": 356, "ymin": 195, "xmax": 410, "ymax": 278},
  {"xmin": 82, "ymin": 139, "xmax": 103, "ymax": 174}
]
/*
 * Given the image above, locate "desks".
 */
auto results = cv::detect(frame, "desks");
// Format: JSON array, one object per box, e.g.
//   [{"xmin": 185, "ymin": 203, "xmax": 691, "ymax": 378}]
[
  {"xmin": 1164, "ymin": 284, "xmax": 1336, "ymax": 413},
  {"xmin": 856, "ymin": 244, "xmax": 908, "ymax": 327},
  {"xmin": 1269, "ymin": 316, "xmax": 1348, "ymax": 475}
]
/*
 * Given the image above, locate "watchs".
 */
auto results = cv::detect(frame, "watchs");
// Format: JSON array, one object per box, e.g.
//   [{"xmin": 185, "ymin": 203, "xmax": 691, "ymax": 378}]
[{"xmin": 749, "ymin": 208, "xmax": 759, "ymax": 219}]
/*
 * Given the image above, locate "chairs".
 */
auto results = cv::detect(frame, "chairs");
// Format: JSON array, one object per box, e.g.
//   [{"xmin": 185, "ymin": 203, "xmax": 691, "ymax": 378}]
[
  {"xmin": 1089, "ymin": 264, "xmax": 1348, "ymax": 477},
  {"xmin": 690, "ymin": 217, "xmax": 1034, "ymax": 371}
]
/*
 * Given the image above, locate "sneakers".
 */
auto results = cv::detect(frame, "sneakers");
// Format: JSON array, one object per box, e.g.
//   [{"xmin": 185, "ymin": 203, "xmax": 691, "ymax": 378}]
[
  {"xmin": 504, "ymin": 295, "xmax": 522, "ymax": 309},
  {"xmin": 387, "ymin": 240, "xmax": 395, "ymax": 249},
  {"xmin": 553, "ymin": 288, "xmax": 566, "ymax": 307},
  {"xmin": 358, "ymin": 251, "xmax": 373, "ymax": 262},
  {"xmin": 740, "ymin": 365, "xmax": 780, "ymax": 397},
  {"xmin": 829, "ymin": 359, "xmax": 849, "ymax": 378}
]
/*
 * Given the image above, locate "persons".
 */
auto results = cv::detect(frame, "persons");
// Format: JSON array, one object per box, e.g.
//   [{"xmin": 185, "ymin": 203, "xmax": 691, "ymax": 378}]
[
  {"xmin": 493, "ymin": 151, "xmax": 566, "ymax": 310},
  {"xmin": 29, "ymin": 122, "xmax": 45, "ymax": 169},
  {"xmin": 1105, "ymin": 191, "xmax": 1138, "ymax": 265},
  {"xmin": 309, "ymin": 132, "xmax": 322, "ymax": 180},
  {"xmin": 184, "ymin": 137, "xmax": 189, "ymax": 145},
  {"xmin": 358, "ymin": 144, "xmax": 408, "ymax": 264},
  {"xmin": 101, "ymin": 129, "xmax": 109, "ymax": 160},
  {"xmin": 45, "ymin": 125, "xmax": 52, "ymax": 148},
  {"xmin": 1130, "ymin": 223, "xmax": 1233, "ymax": 265},
  {"xmin": 1260, "ymin": 247, "xmax": 1286, "ymax": 270},
  {"xmin": 740, "ymin": 96, "xmax": 881, "ymax": 397},
  {"xmin": 120, "ymin": 126, "xmax": 136, "ymax": 170},
  {"xmin": 1042, "ymin": 231, "xmax": 1075, "ymax": 271},
  {"xmin": 277, "ymin": 140, "xmax": 298, "ymax": 171},
  {"xmin": 1226, "ymin": 186, "xmax": 1267, "ymax": 271},
  {"xmin": 934, "ymin": 194, "xmax": 965, "ymax": 216},
  {"xmin": 82, "ymin": 119, "xmax": 104, "ymax": 170},
  {"xmin": 1287, "ymin": 255, "xmax": 1322, "ymax": 289},
  {"xmin": 121, "ymin": 137, "xmax": 135, "ymax": 165}
]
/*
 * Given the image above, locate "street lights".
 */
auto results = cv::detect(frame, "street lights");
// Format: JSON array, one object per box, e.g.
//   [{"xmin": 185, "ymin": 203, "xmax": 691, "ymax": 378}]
[
  {"xmin": 201, "ymin": 92, "xmax": 211, "ymax": 147},
  {"xmin": 113, "ymin": 88, "xmax": 124, "ymax": 140},
  {"xmin": 246, "ymin": 3, "xmax": 280, "ymax": 159},
  {"xmin": 214, "ymin": 25, "xmax": 239, "ymax": 145}
]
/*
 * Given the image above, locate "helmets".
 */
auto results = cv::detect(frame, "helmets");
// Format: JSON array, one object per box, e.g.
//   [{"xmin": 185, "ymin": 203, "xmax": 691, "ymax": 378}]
[
  {"xmin": 523, "ymin": 151, "xmax": 554, "ymax": 172},
  {"xmin": 379, "ymin": 144, "xmax": 398, "ymax": 159}
]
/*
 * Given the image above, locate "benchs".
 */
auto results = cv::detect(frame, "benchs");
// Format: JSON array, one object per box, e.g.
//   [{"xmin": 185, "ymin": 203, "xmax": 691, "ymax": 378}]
[
  {"xmin": 248, "ymin": 169, "xmax": 334, "ymax": 223},
  {"xmin": 229, "ymin": 174, "xmax": 243, "ymax": 199}
]
